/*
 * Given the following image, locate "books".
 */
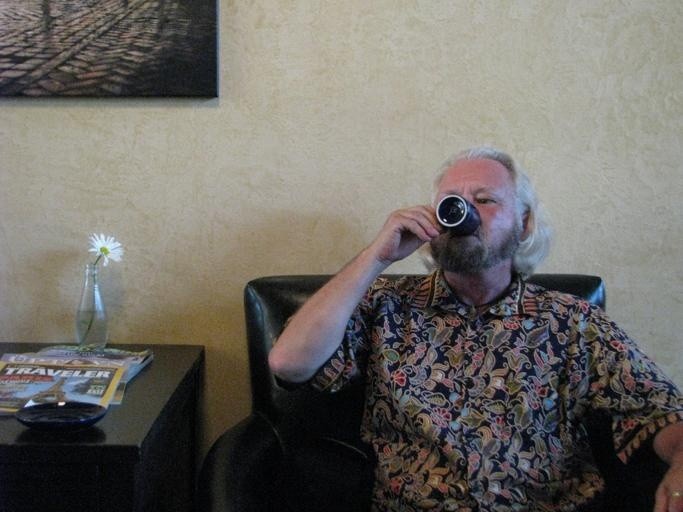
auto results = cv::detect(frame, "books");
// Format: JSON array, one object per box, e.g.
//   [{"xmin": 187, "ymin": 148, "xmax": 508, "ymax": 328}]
[
  {"xmin": 0, "ymin": 348, "xmax": 132, "ymax": 408},
  {"xmin": 0, "ymin": 360, "xmax": 124, "ymax": 415},
  {"xmin": 39, "ymin": 344, "xmax": 153, "ymax": 384}
]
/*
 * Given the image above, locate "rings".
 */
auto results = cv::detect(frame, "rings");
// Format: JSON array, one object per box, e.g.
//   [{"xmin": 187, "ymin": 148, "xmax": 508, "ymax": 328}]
[{"xmin": 668, "ymin": 489, "xmax": 682, "ymax": 499}]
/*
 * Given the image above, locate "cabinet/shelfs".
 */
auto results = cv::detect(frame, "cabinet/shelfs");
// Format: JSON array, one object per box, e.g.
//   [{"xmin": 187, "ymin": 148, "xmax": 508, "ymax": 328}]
[{"xmin": 1, "ymin": 343, "xmax": 204, "ymax": 512}]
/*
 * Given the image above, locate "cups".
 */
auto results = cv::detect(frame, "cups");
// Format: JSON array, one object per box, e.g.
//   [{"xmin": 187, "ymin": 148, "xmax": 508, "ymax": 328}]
[{"xmin": 436, "ymin": 193, "xmax": 483, "ymax": 237}]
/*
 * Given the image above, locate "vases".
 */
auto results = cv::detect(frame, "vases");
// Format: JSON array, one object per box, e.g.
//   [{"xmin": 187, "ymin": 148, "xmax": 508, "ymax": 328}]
[{"xmin": 74, "ymin": 271, "xmax": 107, "ymax": 348}]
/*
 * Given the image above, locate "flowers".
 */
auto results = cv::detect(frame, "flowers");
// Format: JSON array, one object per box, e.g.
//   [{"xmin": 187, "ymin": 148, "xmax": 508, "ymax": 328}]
[{"xmin": 76, "ymin": 235, "xmax": 123, "ymax": 346}]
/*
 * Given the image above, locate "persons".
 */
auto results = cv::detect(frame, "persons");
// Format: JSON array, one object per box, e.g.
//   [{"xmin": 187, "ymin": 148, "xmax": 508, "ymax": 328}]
[{"xmin": 268, "ymin": 149, "xmax": 683, "ymax": 512}]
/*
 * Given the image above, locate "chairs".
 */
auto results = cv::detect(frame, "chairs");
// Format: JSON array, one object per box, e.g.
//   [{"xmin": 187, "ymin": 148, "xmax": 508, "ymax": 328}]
[{"xmin": 202, "ymin": 273, "xmax": 667, "ymax": 512}]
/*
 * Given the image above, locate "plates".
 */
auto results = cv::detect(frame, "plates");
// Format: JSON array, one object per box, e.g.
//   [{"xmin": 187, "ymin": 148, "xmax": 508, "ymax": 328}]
[{"xmin": 12, "ymin": 399, "xmax": 109, "ymax": 431}]
